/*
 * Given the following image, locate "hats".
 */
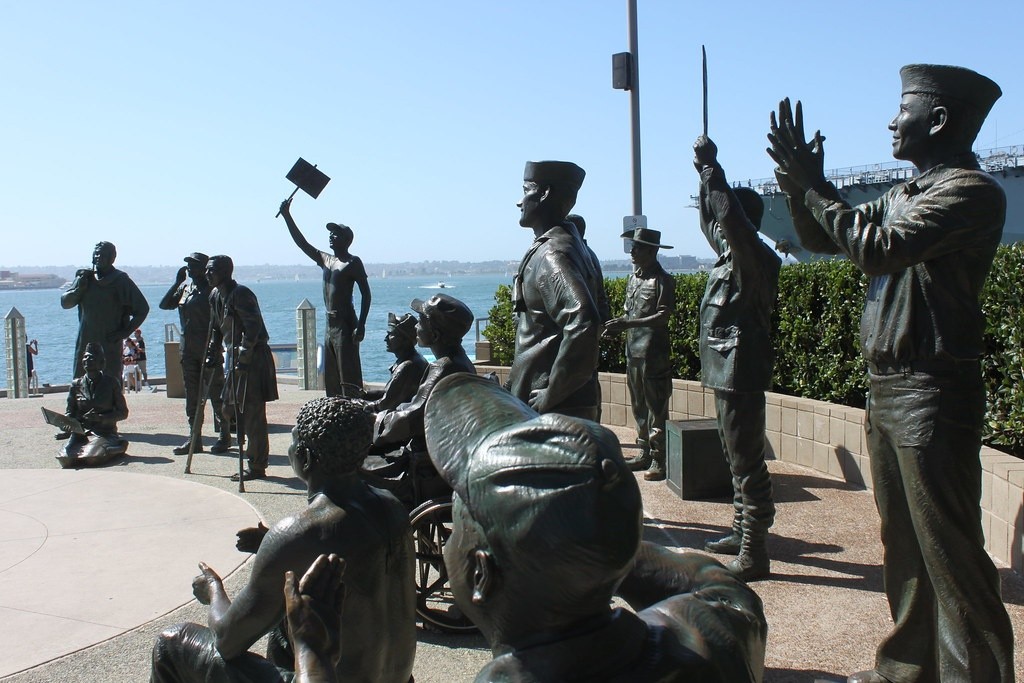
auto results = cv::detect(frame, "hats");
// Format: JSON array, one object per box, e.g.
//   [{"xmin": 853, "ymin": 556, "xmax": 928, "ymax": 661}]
[{"xmin": 136, "ymin": 329, "xmax": 141, "ymax": 335}]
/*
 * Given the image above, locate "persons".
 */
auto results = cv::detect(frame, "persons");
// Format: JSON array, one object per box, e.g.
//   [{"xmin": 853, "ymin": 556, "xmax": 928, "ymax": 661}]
[
  {"xmin": 767, "ymin": 62, "xmax": 1017, "ymax": 683},
  {"xmin": 601, "ymin": 227, "xmax": 677, "ymax": 481},
  {"xmin": 122, "ymin": 329, "xmax": 152, "ymax": 390},
  {"xmin": 732, "ymin": 179, "xmax": 753, "ymax": 187},
  {"xmin": 355, "ymin": 292, "xmax": 478, "ymax": 505},
  {"xmin": 691, "ymin": 132, "xmax": 781, "ymax": 580},
  {"xmin": 159, "ymin": 252, "xmax": 279, "ymax": 481},
  {"xmin": 148, "ymin": 397, "xmax": 414, "ymax": 683},
  {"xmin": 502, "ymin": 158, "xmax": 608, "ymax": 419},
  {"xmin": 54, "ymin": 240, "xmax": 149, "ymax": 469},
  {"xmin": 283, "ymin": 372, "xmax": 767, "ymax": 683},
  {"xmin": 26, "ymin": 335, "xmax": 38, "ymax": 389},
  {"xmin": 278, "ymin": 197, "xmax": 371, "ymax": 399}
]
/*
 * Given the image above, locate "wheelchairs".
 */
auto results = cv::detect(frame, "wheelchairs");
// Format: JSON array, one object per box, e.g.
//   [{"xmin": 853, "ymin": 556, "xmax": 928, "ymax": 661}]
[{"xmin": 408, "ymin": 447, "xmax": 482, "ymax": 635}]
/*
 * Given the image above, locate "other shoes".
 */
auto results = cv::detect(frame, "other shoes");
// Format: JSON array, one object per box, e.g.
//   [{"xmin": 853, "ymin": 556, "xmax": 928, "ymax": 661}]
[
  {"xmin": 145, "ymin": 385, "xmax": 152, "ymax": 390},
  {"xmin": 129, "ymin": 385, "xmax": 135, "ymax": 391}
]
